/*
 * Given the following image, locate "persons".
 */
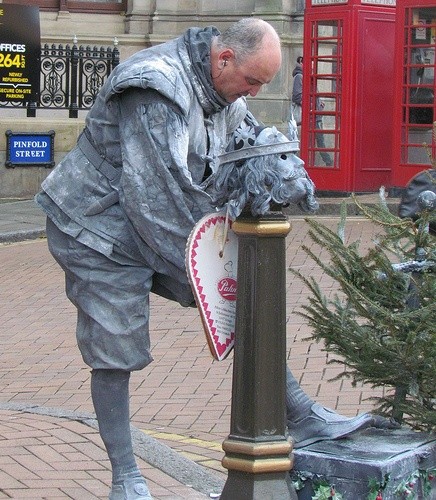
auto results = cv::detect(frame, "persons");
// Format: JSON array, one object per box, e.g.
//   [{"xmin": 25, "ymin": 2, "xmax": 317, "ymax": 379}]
[
  {"xmin": 292, "ymin": 56, "xmax": 334, "ymax": 166},
  {"xmin": 35, "ymin": 18, "xmax": 376, "ymax": 500}
]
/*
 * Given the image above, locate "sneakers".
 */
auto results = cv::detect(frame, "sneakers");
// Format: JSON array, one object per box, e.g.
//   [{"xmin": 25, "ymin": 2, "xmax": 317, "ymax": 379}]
[{"xmin": 325, "ymin": 160, "xmax": 335, "ymax": 167}]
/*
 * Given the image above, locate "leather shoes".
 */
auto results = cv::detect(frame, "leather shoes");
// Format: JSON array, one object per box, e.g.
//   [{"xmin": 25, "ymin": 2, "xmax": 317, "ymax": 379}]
[
  {"xmin": 287, "ymin": 403, "xmax": 377, "ymax": 449},
  {"xmin": 109, "ymin": 476, "xmax": 153, "ymax": 500}
]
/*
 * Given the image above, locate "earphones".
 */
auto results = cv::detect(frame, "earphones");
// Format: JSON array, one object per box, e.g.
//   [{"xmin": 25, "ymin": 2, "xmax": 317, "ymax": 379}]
[{"xmin": 223, "ymin": 56, "xmax": 228, "ymax": 65}]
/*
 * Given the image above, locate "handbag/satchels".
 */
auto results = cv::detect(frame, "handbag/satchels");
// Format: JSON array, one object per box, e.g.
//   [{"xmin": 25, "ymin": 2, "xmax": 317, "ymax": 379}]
[{"xmin": 290, "ymin": 101, "xmax": 302, "ymax": 125}]
